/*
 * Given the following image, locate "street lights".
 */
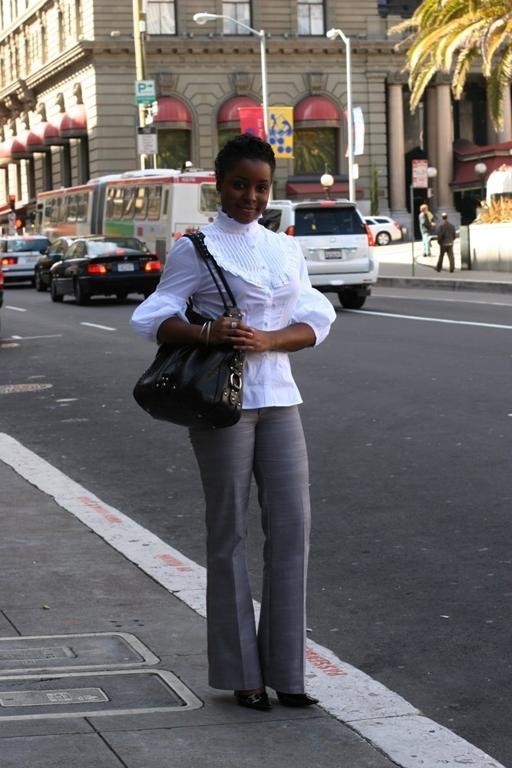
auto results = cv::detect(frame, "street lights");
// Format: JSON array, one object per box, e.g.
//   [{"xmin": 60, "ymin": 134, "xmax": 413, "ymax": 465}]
[
  {"xmin": 192, "ymin": 12, "xmax": 273, "ymax": 201},
  {"xmin": 326, "ymin": 28, "xmax": 356, "ymax": 201}
]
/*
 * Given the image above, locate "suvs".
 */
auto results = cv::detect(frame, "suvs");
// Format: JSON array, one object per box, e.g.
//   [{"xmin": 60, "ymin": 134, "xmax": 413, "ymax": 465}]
[
  {"xmin": 365, "ymin": 215, "xmax": 402, "ymax": 246},
  {"xmin": 255, "ymin": 198, "xmax": 379, "ymax": 308}
]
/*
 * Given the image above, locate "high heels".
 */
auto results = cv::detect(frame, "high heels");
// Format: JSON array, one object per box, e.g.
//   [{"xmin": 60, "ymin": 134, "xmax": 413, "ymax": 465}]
[{"xmin": 233, "ymin": 683, "xmax": 319, "ymax": 714}]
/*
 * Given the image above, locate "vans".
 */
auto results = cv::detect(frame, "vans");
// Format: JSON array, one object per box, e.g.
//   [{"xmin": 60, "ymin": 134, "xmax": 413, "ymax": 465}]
[{"xmin": 1, "ymin": 234, "xmax": 52, "ymax": 289}]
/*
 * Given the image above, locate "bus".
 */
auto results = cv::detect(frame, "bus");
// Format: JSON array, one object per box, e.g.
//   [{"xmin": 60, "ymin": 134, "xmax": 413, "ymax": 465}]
[{"xmin": 33, "ymin": 167, "xmax": 222, "ymax": 277}]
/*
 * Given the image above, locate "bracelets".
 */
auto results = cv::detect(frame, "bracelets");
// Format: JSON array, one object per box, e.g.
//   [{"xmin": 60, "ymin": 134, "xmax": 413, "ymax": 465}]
[{"xmin": 200, "ymin": 321, "xmax": 211, "ymax": 348}]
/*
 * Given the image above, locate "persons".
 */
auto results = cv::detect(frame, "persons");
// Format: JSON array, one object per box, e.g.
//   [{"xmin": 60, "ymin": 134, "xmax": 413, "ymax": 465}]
[
  {"xmin": 418, "ymin": 204, "xmax": 437, "ymax": 257},
  {"xmin": 437, "ymin": 213, "xmax": 455, "ymax": 272},
  {"xmin": 130, "ymin": 133, "xmax": 337, "ymax": 712}
]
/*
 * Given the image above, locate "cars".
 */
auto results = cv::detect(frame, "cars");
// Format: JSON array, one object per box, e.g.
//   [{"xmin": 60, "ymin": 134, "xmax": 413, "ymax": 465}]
[{"xmin": 32, "ymin": 234, "xmax": 163, "ymax": 306}]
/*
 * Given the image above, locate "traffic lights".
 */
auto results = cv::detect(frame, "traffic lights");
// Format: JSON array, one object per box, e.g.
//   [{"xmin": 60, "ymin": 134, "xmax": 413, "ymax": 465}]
[
  {"xmin": 8, "ymin": 195, "xmax": 16, "ymax": 210},
  {"xmin": 15, "ymin": 216, "xmax": 25, "ymax": 229}
]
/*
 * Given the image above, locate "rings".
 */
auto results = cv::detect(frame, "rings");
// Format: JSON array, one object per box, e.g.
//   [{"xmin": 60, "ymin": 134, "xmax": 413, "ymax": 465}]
[{"xmin": 232, "ymin": 321, "xmax": 237, "ymax": 328}]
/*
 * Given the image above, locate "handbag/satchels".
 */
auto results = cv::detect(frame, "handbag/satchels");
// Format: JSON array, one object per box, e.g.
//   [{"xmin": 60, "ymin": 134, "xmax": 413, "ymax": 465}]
[{"xmin": 132, "ymin": 304, "xmax": 248, "ymax": 430}]
